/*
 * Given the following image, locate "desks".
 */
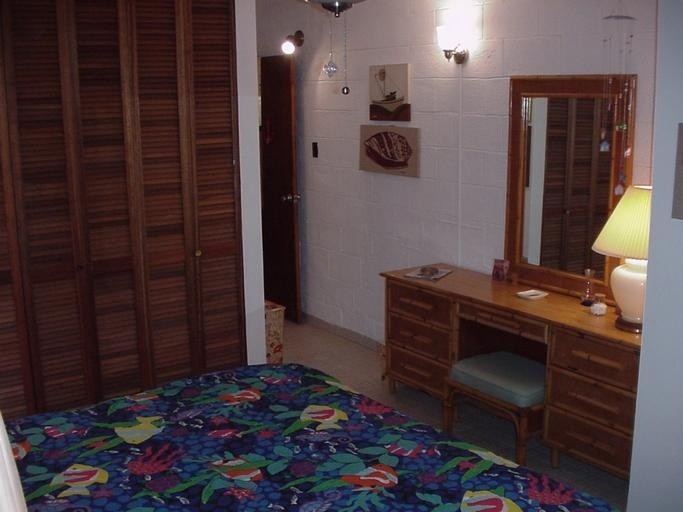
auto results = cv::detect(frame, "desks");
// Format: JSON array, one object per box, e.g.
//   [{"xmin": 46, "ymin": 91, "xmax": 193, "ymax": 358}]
[{"xmin": 378, "ymin": 263, "xmax": 663, "ymax": 484}]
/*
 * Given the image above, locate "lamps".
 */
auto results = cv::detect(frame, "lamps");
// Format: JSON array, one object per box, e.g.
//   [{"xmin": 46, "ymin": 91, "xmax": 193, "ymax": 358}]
[
  {"xmin": 590, "ymin": 183, "xmax": 653, "ymax": 333},
  {"xmin": 281, "ymin": 28, "xmax": 305, "ymax": 57},
  {"xmin": 319, "ymin": 0, "xmax": 351, "ymax": 96},
  {"xmin": 436, "ymin": 25, "xmax": 467, "ymax": 65}
]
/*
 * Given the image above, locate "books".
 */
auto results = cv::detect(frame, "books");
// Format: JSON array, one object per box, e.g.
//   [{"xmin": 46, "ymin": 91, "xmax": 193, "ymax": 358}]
[{"xmin": 403, "ymin": 266, "xmax": 452, "ymax": 280}]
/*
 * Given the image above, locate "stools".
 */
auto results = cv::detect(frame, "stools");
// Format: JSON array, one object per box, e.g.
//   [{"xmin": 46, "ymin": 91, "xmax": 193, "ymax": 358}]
[{"xmin": 438, "ymin": 352, "xmax": 550, "ymax": 471}]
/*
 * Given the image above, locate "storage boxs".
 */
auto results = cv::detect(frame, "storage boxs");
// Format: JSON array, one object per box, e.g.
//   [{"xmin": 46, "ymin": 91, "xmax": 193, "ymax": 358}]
[{"xmin": 264, "ymin": 300, "xmax": 287, "ymax": 366}]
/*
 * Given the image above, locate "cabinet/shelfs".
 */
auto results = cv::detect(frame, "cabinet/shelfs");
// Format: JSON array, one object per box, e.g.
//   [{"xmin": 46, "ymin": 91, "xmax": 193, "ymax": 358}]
[
  {"xmin": 539, "ymin": 90, "xmax": 615, "ymax": 283},
  {"xmin": 0, "ymin": 0, "xmax": 248, "ymax": 427}
]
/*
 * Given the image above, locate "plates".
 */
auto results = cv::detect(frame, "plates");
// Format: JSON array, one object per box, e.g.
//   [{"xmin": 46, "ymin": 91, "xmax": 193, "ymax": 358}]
[{"xmin": 517, "ymin": 289, "xmax": 548, "ymax": 300}]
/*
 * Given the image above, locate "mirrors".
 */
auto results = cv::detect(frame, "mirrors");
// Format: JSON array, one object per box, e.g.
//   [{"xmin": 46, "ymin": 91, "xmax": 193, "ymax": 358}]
[
  {"xmin": 518, "ymin": 92, "xmax": 620, "ymax": 285},
  {"xmin": 505, "ymin": 72, "xmax": 637, "ymax": 307}
]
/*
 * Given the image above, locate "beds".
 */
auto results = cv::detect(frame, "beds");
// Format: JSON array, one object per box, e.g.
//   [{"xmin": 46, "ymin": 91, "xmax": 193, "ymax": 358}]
[{"xmin": 0, "ymin": 360, "xmax": 621, "ymax": 512}]
